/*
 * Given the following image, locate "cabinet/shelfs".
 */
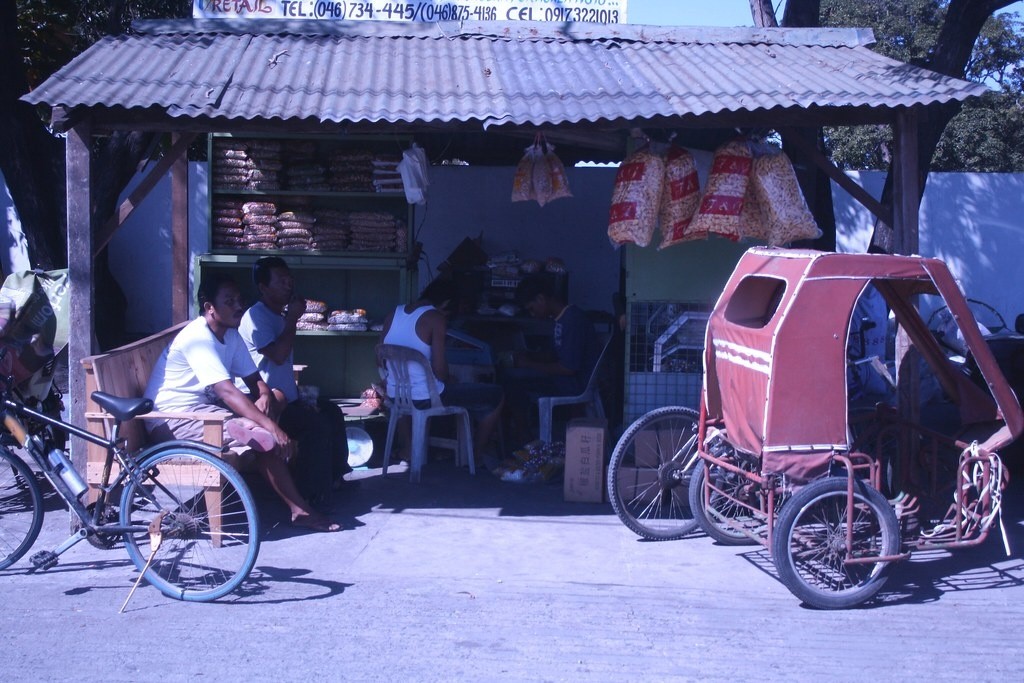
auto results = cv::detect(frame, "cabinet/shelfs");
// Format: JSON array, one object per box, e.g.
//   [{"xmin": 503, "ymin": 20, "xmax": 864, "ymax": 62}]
[
  {"xmin": 446, "ymin": 315, "xmax": 612, "ymax": 386},
  {"xmin": 194, "ymin": 133, "xmax": 417, "ymax": 426}
]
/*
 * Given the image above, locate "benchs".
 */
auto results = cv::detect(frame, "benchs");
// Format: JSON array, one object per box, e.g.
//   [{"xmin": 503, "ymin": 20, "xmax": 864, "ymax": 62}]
[{"xmin": 80, "ymin": 320, "xmax": 253, "ymax": 548}]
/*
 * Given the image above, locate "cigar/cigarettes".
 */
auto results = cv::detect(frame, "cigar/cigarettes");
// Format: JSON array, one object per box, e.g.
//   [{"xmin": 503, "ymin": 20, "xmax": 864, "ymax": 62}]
[{"xmin": 286, "ymin": 457, "xmax": 289, "ymax": 464}]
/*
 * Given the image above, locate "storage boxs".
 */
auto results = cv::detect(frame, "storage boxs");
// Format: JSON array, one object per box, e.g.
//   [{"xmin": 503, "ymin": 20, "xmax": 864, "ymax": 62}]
[
  {"xmin": 660, "ymin": 428, "xmax": 697, "ymax": 467},
  {"xmin": 633, "ymin": 430, "xmax": 660, "ymax": 466},
  {"xmin": 671, "ymin": 468, "xmax": 693, "ymax": 504},
  {"xmin": 604, "ymin": 461, "xmax": 637, "ymax": 507},
  {"xmin": 637, "ymin": 467, "xmax": 666, "ymax": 505},
  {"xmin": 563, "ymin": 416, "xmax": 608, "ymax": 504}
]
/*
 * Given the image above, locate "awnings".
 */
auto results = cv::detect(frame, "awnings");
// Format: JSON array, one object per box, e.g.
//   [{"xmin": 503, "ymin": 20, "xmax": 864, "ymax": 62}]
[{"xmin": 19, "ymin": 34, "xmax": 987, "ymax": 131}]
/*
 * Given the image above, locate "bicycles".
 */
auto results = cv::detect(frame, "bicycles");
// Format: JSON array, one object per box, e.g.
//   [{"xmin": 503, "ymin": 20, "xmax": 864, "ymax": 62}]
[
  {"xmin": 1, "ymin": 377, "xmax": 259, "ymax": 616},
  {"xmin": 606, "ymin": 318, "xmax": 882, "ymax": 542}
]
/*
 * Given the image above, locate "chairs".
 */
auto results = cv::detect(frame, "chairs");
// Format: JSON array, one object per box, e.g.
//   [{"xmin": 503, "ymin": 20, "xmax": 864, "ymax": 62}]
[
  {"xmin": 376, "ymin": 343, "xmax": 477, "ymax": 484},
  {"xmin": 535, "ymin": 320, "xmax": 615, "ymax": 456}
]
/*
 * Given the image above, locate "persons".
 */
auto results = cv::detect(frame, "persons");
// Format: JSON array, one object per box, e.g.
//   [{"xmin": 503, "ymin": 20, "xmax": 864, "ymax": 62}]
[
  {"xmin": 140, "ymin": 273, "xmax": 340, "ymax": 532},
  {"xmin": 377, "ymin": 275, "xmax": 599, "ymax": 472},
  {"xmin": 235, "ymin": 257, "xmax": 362, "ymax": 512}
]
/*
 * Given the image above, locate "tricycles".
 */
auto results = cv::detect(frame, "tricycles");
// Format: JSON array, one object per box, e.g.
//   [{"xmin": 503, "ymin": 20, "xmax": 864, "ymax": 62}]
[{"xmin": 689, "ymin": 245, "xmax": 1023, "ymax": 611}]
